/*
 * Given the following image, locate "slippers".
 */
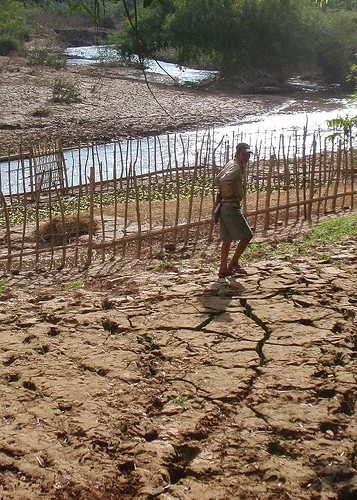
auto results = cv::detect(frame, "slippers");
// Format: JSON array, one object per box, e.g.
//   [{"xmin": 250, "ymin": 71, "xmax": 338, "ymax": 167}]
[
  {"xmin": 218, "ymin": 269, "xmax": 234, "ymax": 278},
  {"xmin": 229, "ymin": 264, "xmax": 247, "ymax": 274}
]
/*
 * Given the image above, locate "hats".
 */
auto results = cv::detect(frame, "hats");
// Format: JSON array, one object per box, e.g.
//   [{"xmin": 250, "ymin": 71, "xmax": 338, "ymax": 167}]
[{"xmin": 236, "ymin": 142, "xmax": 252, "ymax": 153}]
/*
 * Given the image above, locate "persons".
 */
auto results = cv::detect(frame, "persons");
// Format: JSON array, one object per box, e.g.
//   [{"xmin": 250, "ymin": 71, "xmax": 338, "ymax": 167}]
[{"xmin": 215, "ymin": 143, "xmax": 255, "ymax": 276}]
